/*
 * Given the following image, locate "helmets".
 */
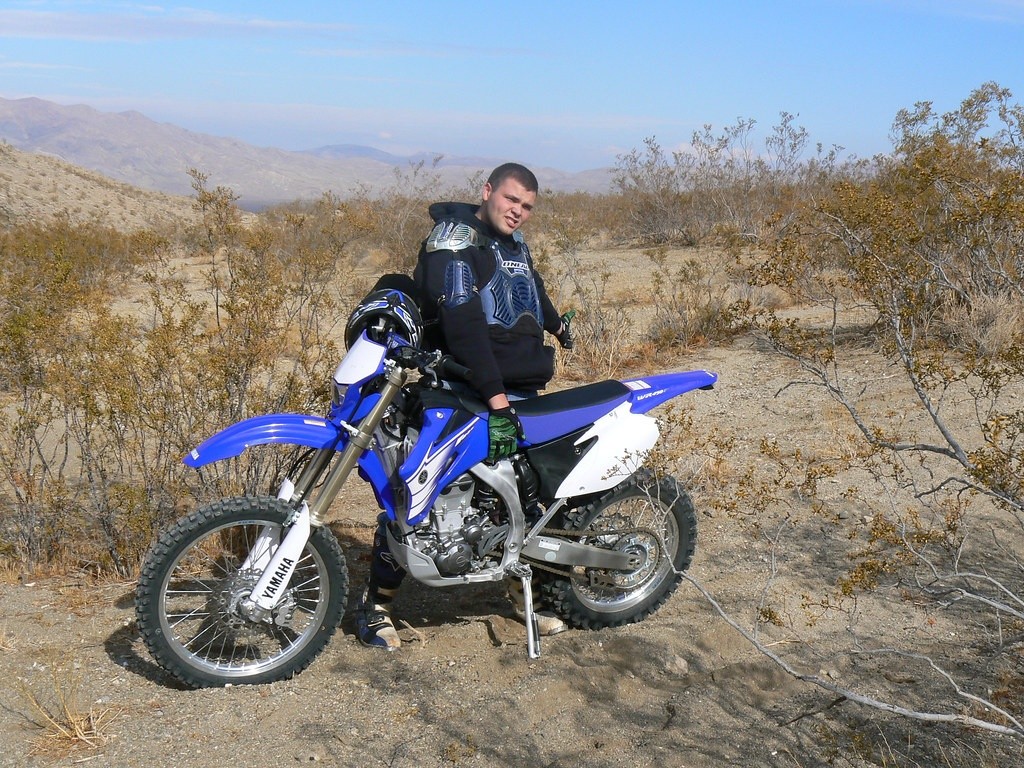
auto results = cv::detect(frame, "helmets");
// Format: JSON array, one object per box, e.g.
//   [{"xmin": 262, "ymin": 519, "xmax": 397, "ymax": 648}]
[{"xmin": 344, "ymin": 274, "xmax": 424, "ymax": 354}]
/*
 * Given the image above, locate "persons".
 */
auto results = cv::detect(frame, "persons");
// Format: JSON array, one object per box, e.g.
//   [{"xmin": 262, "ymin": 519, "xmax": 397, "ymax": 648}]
[{"xmin": 354, "ymin": 163, "xmax": 575, "ymax": 652}]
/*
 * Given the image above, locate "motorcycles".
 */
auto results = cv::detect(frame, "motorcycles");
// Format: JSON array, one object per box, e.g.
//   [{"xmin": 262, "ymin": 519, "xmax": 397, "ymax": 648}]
[{"xmin": 132, "ymin": 288, "xmax": 718, "ymax": 691}]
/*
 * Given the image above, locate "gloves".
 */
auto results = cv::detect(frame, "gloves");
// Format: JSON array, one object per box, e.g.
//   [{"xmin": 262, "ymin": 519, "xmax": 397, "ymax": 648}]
[
  {"xmin": 554, "ymin": 312, "xmax": 575, "ymax": 349},
  {"xmin": 483, "ymin": 406, "xmax": 526, "ymax": 463}
]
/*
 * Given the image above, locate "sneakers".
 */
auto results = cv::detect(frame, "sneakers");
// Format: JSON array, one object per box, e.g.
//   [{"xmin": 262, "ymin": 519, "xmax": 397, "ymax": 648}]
[
  {"xmin": 508, "ymin": 576, "xmax": 569, "ymax": 635},
  {"xmin": 355, "ymin": 576, "xmax": 401, "ymax": 651}
]
